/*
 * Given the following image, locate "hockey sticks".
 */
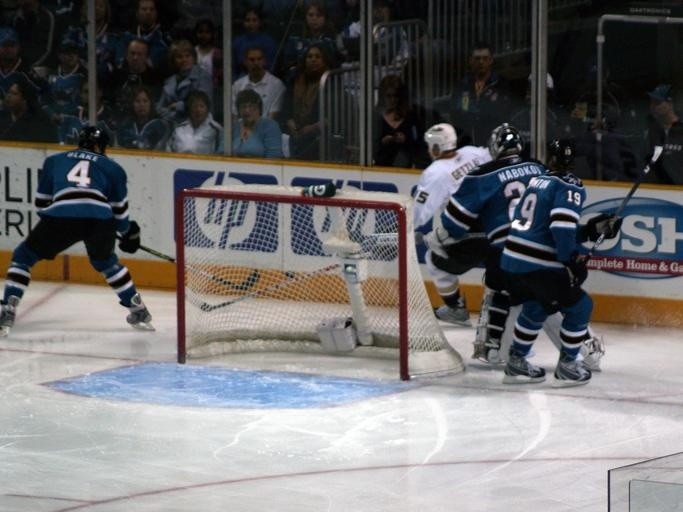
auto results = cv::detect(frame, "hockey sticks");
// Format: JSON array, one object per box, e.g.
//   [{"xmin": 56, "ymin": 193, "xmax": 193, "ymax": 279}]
[
  {"xmin": 582, "ymin": 116, "xmax": 667, "ymax": 264},
  {"xmin": 116, "ymin": 233, "xmax": 260, "ymax": 290},
  {"xmin": 185, "ymin": 265, "xmax": 344, "ymax": 312}
]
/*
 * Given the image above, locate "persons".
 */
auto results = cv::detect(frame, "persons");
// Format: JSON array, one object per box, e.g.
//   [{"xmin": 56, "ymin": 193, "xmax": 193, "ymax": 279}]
[
  {"xmin": 0, "ymin": 2, "xmax": 682, "ymax": 184},
  {"xmin": 498, "ymin": 130, "xmax": 624, "ymax": 382},
  {"xmin": 0, "ymin": 125, "xmax": 154, "ymax": 329},
  {"xmin": 426, "ymin": 123, "xmax": 606, "ymax": 368},
  {"xmin": 409, "ymin": 121, "xmax": 493, "ymax": 324}
]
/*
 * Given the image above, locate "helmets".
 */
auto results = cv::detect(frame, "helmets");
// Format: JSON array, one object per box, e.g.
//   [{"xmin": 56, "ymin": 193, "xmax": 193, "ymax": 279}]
[
  {"xmin": 488, "ymin": 122, "xmax": 524, "ymax": 160},
  {"xmin": 78, "ymin": 126, "xmax": 114, "ymax": 156},
  {"xmin": 547, "ymin": 138, "xmax": 584, "ymax": 175},
  {"xmin": 424, "ymin": 122, "xmax": 458, "ymax": 160}
]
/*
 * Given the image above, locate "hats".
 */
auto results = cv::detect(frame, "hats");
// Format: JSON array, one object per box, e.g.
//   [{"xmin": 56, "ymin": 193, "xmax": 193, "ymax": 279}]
[{"xmin": 646, "ymin": 86, "xmax": 677, "ymax": 103}]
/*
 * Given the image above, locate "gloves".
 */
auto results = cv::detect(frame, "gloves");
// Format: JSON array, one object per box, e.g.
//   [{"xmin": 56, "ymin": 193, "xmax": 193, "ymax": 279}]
[
  {"xmin": 118, "ymin": 220, "xmax": 141, "ymax": 254},
  {"xmin": 564, "ymin": 251, "xmax": 588, "ymax": 290},
  {"xmin": 425, "ymin": 216, "xmax": 460, "ymax": 247},
  {"xmin": 587, "ymin": 213, "xmax": 623, "ymax": 242}
]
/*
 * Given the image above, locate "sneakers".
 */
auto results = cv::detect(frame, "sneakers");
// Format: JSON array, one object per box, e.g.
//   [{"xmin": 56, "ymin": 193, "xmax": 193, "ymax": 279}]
[
  {"xmin": 479, "ymin": 340, "xmax": 606, "ymax": 382},
  {"xmin": 435, "ymin": 298, "xmax": 470, "ymax": 322}
]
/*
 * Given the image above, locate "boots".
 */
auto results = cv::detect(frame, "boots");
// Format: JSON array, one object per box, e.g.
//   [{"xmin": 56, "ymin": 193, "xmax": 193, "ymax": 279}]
[
  {"xmin": 118, "ymin": 292, "xmax": 152, "ymax": 325},
  {"xmin": 0, "ymin": 295, "xmax": 20, "ymax": 327}
]
